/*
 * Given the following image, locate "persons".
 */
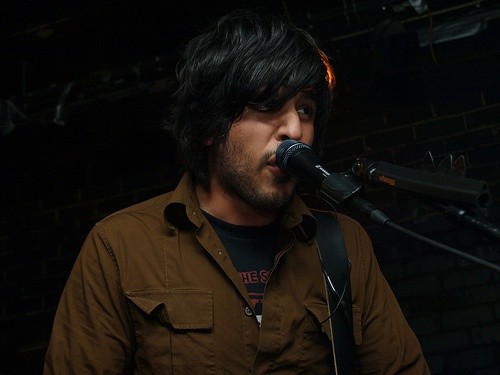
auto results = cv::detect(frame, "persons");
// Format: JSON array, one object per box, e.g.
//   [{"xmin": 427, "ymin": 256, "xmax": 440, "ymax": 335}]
[{"xmin": 41, "ymin": 17, "xmax": 432, "ymax": 375}]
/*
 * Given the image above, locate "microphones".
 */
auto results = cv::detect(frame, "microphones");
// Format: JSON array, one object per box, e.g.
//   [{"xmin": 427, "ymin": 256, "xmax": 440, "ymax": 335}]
[{"xmin": 276, "ymin": 140, "xmax": 390, "ymax": 225}]
